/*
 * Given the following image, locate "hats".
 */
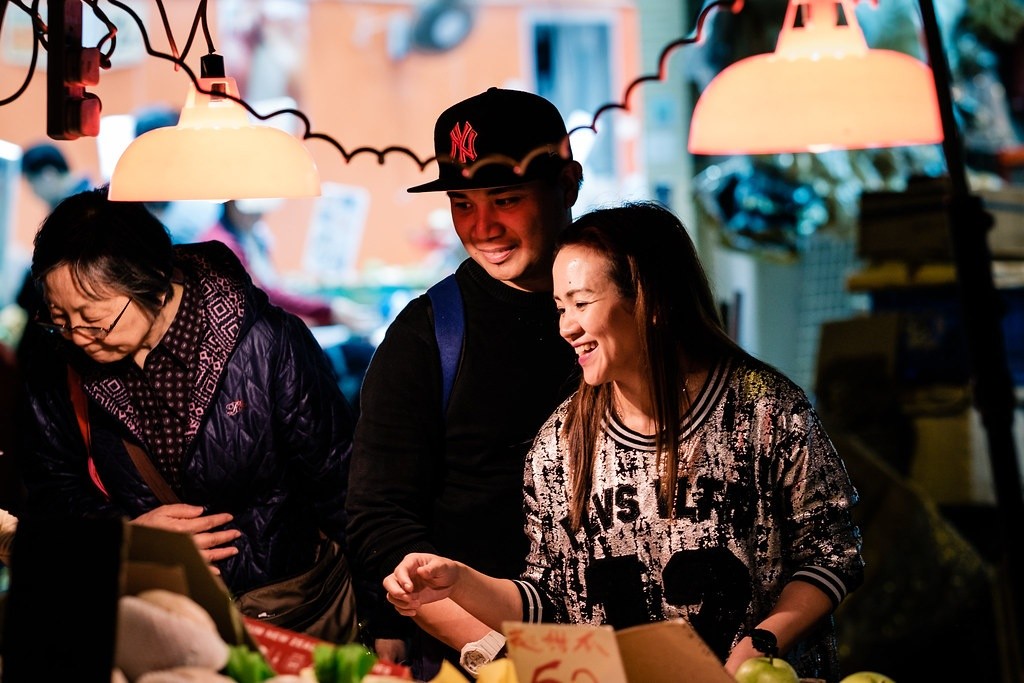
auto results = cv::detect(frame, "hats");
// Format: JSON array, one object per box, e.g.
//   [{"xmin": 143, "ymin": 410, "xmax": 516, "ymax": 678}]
[{"xmin": 407, "ymin": 86, "xmax": 587, "ymax": 192}]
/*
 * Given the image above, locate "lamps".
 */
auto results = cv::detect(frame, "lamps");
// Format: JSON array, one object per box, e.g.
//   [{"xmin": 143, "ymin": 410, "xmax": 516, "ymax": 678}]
[
  {"xmin": 107, "ymin": 54, "xmax": 321, "ymax": 203},
  {"xmin": 687, "ymin": 0, "xmax": 944, "ymax": 155}
]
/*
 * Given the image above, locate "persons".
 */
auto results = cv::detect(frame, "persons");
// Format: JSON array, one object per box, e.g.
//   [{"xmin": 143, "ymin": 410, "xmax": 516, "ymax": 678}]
[
  {"xmin": 340, "ymin": 88, "xmax": 583, "ymax": 681},
  {"xmin": 21, "ymin": 146, "xmax": 94, "ymax": 207},
  {"xmin": 9, "ymin": 193, "xmax": 366, "ymax": 649},
  {"xmin": 199, "ymin": 201, "xmax": 358, "ymax": 329},
  {"xmin": 382, "ymin": 201, "xmax": 865, "ymax": 683}
]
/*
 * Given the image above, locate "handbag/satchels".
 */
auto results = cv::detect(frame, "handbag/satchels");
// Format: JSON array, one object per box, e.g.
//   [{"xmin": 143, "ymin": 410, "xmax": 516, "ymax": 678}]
[{"xmin": 236, "ymin": 549, "xmax": 361, "ymax": 645}]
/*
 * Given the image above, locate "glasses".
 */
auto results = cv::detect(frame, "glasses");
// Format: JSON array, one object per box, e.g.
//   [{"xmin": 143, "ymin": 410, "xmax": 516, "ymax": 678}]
[{"xmin": 34, "ymin": 296, "xmax": 132, "ymax": 344}]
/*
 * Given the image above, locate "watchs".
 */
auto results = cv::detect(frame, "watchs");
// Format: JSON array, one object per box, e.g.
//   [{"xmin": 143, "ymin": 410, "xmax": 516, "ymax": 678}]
[
  {"xmin": 459, "ymin": 630, "xmax": 506, "ymax": 679},
  {"xmin": 745, "ymin": 628, "xmax": 779, "ymax": 658}
]
